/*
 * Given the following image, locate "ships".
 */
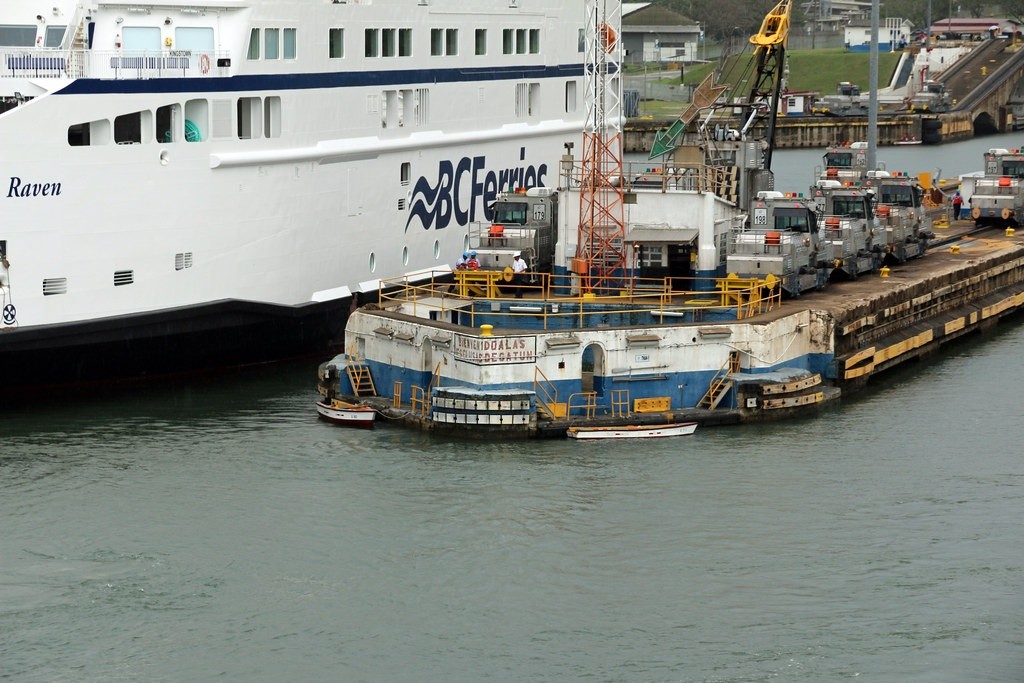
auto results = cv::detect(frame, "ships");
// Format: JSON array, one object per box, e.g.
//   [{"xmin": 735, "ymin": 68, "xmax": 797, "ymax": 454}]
[{"xmin": 0, "ymin": 0, "xmax": 624, "ymax": 337}]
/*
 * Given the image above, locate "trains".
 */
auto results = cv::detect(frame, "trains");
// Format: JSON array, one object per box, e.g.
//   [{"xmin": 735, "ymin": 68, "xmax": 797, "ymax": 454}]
[
  {"xmin": 726, "ymin": 141, "xmax": 1023, "ymax": 304},
  {"xmin": 461, "ymin": 183, "xmax": 559, "ymax": 294},
  {"xmin": 907, "ymin": 79, "xmax": 953, "ymax": 113},
  {"xmin": 813, "ymin": 79, "xmax": 881, "ymax": 116}
]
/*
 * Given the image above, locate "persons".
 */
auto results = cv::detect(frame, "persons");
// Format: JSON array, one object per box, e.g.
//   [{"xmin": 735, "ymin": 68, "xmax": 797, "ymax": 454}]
[
  {"xmin": 447, "ymin": 251, "xmax": 480, "ymax": 295},
  {"xmin": 952, "ymin": 189, "xmax": 964, "ymax": 221},
  {"xmin": 511, "ymin": 253, "xmax": 527, "ymax": 297}
]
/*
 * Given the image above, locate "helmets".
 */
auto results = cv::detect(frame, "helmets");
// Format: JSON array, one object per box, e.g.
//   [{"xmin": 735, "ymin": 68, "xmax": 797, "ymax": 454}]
[
  {"xmin": 471, "ymin": 251, "xmax": 476, "ymax": 256},
  {"xmin": 463, "ymin": 252, "xmax": 469, "ymax": 258},
  {"xmin": 956, "ymin": 189, "xmax": 961, "ymax": 193}
]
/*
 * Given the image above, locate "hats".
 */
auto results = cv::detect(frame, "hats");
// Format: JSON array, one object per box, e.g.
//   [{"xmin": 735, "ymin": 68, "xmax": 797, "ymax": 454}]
[{"xmin": 513, "ymin": 253, "xmax": 520, "ymax": 258}]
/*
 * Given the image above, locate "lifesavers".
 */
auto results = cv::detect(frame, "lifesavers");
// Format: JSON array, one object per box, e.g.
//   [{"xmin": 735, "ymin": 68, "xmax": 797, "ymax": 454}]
[{"xmin": 198, "ymin": 54, "xmax": 210, "ymax": 75}]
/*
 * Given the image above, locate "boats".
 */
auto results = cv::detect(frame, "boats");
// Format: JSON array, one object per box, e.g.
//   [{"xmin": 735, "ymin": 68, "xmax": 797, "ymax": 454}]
[
  {"xmin": 317, "ymin": 397, "xmax": 377, "ymax": 424},
  {"xmin": 567, "ymin": 421, "xmax": 698, "ymax": 439}
]
